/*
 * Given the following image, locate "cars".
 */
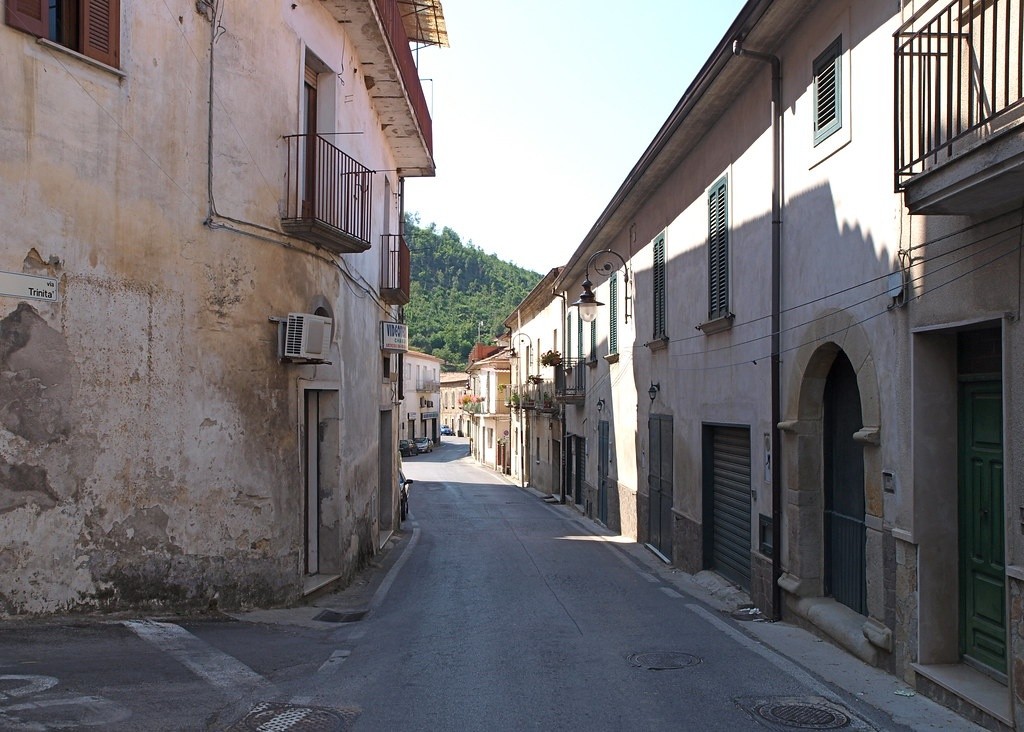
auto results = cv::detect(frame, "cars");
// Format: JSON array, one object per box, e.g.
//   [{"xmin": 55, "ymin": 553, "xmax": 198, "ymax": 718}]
[
  {"xmin": 440, "ymin": 425, "xmax": 451, "ymax": 435},
  {"xmin": 400, "ymin": 439, "xmax": 419, "ymax": 457},
  {"xmin": 413, "ymin": 437, "xmax": 434, "ymax": 454},
  {"xmin": 400, "ymin": 468, "xmax": 413, "ymax": 521}
]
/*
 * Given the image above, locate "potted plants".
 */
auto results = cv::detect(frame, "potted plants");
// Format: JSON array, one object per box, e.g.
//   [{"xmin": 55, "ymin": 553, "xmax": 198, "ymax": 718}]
[
  {"xmin": 511, "ymin": 390, "xmax": 520, "ymax": 408},
  {"xmin": 504, "ymin": 395, "xmax": 511, "ymax": 407},
  {"xmin": 497, "ymin": 436, "xmax": 509, "ymax": 446},
  {"xmin": 544, "ymin": 392, "xmax": 553, "ymax": 408},
  {"xmin": 462, "ymin": 402, "xmax": 482, "ymax": 415},
  {"xmin": 541, "ymin": 349, "xmax": 564, "ymax": 368},
  {"xmin": 497, "ymin": 384, "xmax": 507, "ymax": 392},
  {"xmin": 523, "ymin": 392, "xmax": 534, "ymax": 405},
  {"xmin": 527, "ymin": 375, "xmax": 544, "ymax": 384}
]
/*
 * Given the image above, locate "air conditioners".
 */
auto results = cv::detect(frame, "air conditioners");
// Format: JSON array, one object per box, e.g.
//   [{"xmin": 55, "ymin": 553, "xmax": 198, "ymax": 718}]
[
  {"xmin": 422, "ymin": 398, "xmax": 427, "ymax": 407},
  {"xmin": 285, "ymin": 312, "xmax": 333, "ymax": 360},
  {"xmin": 428, "ymin": 401, "xmax": 434, "ymax": 407}
]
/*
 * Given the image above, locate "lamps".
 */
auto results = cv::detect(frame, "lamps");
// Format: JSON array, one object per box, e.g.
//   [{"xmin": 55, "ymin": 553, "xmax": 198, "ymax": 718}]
[
  {"xmin": 571, "ymin": 248, "xmax": 633, "ymax": 325},
  {"xmin": 510, "ymin": 332, "xmax": 532, "ymax": 357},
  {"xmin": 649, "ymin": 380, "xmax": 661, "ymax": 404},
  {"xmin": 597, "ymin": 396, "xmax": 606, "ymax": 413}
]
